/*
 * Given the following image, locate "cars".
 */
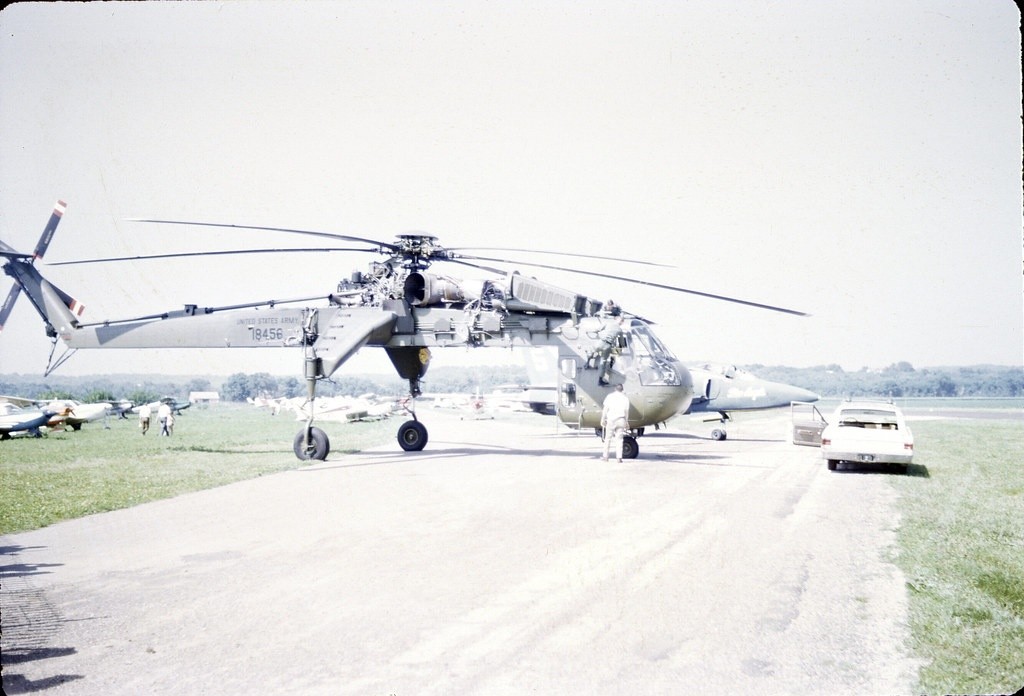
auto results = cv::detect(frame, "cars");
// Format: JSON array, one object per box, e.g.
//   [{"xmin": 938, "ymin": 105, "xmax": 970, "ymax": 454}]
[{"xmin": 789, "ymin": 397, "xmax": 916, "ymax": 474}]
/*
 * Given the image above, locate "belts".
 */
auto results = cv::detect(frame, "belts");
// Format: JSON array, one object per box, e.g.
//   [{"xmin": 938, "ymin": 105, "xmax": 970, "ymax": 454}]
[{"xmin": 602, "ymin": 339, "xmax": 612, "ymax": 345}]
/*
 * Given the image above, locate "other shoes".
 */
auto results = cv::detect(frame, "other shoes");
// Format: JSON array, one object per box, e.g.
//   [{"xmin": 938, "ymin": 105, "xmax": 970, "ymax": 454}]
[
  {"xmin": 618, "ymin": 459, "xmax": 622, "ymax": 463},
  {"xmin": 600, "ymin": 457, "xmax": 609, "ymax": 461}
]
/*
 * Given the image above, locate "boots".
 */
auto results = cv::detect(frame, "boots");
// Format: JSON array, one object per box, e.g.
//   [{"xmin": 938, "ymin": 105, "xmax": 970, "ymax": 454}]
[
  {"xmin": 584, "ymin": 359, "xmax": 590, "ymax": 369},
  {"xmin": 599, "ymin": 377, "xmax": 609, "ymax": 385}
]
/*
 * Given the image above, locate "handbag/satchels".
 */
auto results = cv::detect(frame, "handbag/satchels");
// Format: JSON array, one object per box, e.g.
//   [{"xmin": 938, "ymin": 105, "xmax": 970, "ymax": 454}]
[{"xmin": 138, "ymin": 421, "xmax": 143, "ymax": 428}]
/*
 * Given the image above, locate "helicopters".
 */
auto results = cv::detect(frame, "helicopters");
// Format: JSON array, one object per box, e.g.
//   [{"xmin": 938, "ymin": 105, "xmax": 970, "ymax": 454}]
[{"xmin": 2, "ymin": 197, "xmax": 814, "ymax": 461}]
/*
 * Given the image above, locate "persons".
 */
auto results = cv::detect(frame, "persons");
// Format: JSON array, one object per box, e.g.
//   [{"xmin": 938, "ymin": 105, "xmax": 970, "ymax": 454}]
[
  {"xmin": 600, "ymin": 383, "xmax": 629, "ymax": 461},
  {"xmin": 155, "ymin": 401, "xmax": 175, "ymax": 436},
  {"xmin": 138, "ymin": 402, "xmax": 150, "ymax": 437}
]
